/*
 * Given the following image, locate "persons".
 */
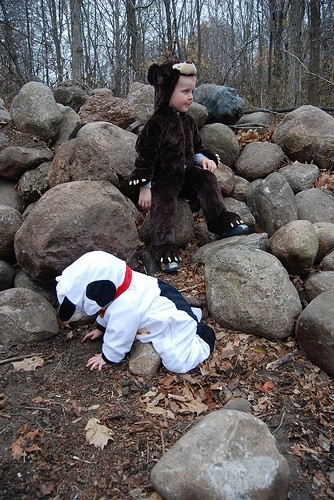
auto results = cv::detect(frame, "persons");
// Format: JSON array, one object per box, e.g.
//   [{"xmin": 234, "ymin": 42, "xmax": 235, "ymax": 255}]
[
  {"xmin": 134, "ymin": 60, "xmax": 250, "ymax": 273},
  {"xmin": 55, "ymin": 251, "xmax": 216, "ymax": 372}
]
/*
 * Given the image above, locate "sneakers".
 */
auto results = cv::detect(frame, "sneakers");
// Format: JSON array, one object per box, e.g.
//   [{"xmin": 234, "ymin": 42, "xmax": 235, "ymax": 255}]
[
  {"xmin": 216, "ymin": 223, "xmax": 249, "ymax": 239},
  {"xmin": 161, "ymin": 262, "xmax": 180, "ymax": 273}
]
